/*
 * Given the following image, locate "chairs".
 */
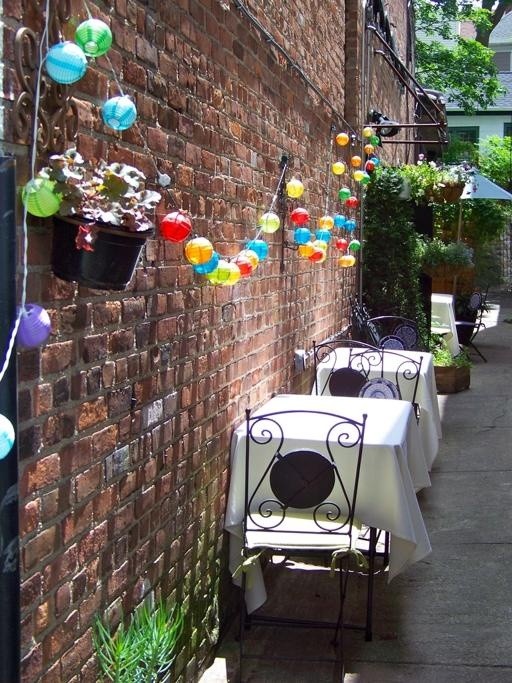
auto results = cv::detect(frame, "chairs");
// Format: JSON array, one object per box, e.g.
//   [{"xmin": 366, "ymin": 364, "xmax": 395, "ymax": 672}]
[
  {"xmin": 451, "ymin": 290, "xmax": 491, "ymax": 360},
  {"xmin": 311, "ymin": 338, "xmax": 383, "ymax": 397},
  {"xmin": 346, "ymin": 346, "xmax": 427, "ymax": 426},
  {"xmin": 365, "ymin": 314, "xmax": 421, "ymax": 349},
  {"xmin": 241, "ymin": 406, "xmax": 390, "ymax": 681}
]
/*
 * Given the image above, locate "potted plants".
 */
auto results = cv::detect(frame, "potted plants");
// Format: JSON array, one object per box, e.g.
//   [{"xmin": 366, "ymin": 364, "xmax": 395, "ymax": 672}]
[
  {"xmin": 439, "ymin": 167, "xmax": 468, "ymax": 201},
  {"xmin": 42, "ymin": 145, "xmax": 162, "ymax": 292},
  {"xmin": 423, "ymin": 341, "xmax": 475, "ymax": 393}
]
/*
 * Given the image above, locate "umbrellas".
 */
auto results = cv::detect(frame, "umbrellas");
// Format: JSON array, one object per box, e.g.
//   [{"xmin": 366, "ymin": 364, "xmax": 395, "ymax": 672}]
[{"xmin": 448, "ymin": 159, "xmax": 511, "ymax": 296}]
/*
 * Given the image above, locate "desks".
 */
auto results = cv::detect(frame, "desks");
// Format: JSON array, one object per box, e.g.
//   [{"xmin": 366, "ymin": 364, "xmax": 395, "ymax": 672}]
[
  {"xmin": 313, "ymin": 346, "xmax": 441, "ymax": 476},
  {"xmin": 425, "ymin": 295, "xmax": 457, "ymax": 359},
  {"xmin": 224, "ymin": 393, "xmax": 434, "ymax": 643}
]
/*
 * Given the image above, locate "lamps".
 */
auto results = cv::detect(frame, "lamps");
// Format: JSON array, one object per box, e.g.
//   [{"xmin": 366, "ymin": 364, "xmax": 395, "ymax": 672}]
[{"xmin": 369, "ymin": 109, "xmax": 402, "ymax": 135}]
